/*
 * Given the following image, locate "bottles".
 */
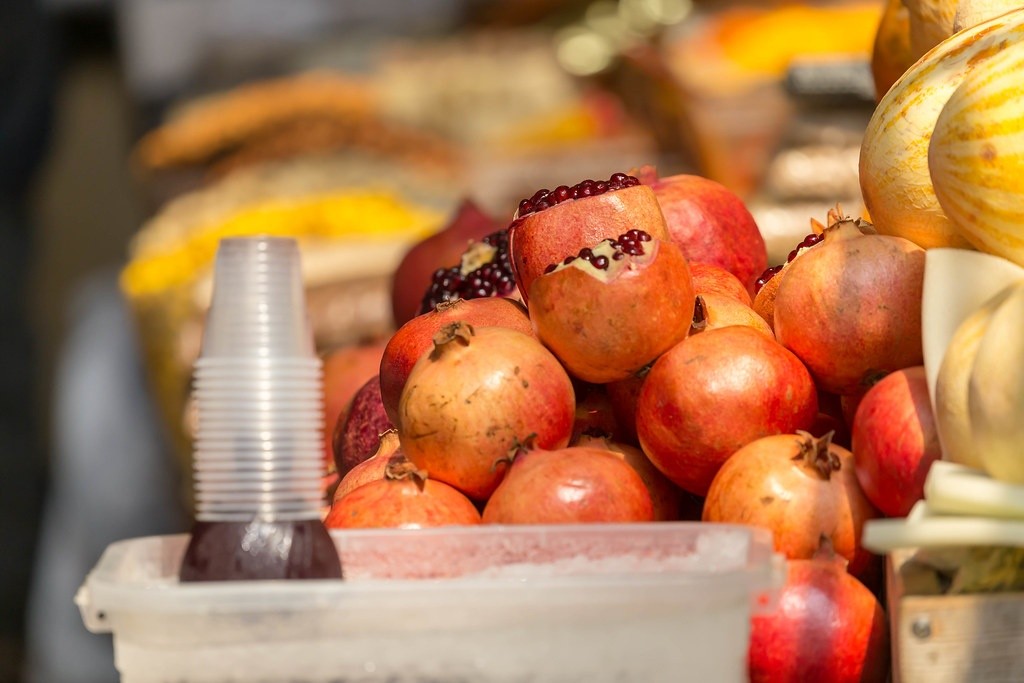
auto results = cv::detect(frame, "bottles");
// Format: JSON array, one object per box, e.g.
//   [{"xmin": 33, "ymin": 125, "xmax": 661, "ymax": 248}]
[{"xmin": 178, "ymin": 503, "xmax": 342, "ymax": 587}]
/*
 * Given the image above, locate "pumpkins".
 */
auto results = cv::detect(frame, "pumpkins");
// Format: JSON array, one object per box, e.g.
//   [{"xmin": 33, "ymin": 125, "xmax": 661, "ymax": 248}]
[{"xmin": 935, "ymin": 278, "xmax": 1024, "ymax": 490}]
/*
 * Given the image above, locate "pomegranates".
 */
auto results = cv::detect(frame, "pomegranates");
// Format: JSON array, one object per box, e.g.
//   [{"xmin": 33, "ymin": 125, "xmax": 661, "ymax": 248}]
[{"xmin": 329, "ymin": 165, "xmax": 935, "ymax": 683}]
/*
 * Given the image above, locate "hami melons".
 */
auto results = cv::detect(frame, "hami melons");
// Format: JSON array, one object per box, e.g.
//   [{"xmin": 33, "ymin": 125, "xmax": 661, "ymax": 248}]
[{"xmin": 855, "ymin": 0, "xmax": 1024, "ymax": 266}]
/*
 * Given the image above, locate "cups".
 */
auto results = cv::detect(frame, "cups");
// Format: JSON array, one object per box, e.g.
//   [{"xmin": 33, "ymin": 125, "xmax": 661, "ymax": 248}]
[{"xmin": 189, "ymin": 232, "xmax": 333, "ymax": 525}]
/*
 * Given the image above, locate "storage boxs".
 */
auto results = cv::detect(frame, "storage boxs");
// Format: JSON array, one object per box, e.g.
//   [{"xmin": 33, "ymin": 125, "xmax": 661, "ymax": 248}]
[{"xmin": 75, "ymin": 525, "xmax": 787, "ymax": 683}]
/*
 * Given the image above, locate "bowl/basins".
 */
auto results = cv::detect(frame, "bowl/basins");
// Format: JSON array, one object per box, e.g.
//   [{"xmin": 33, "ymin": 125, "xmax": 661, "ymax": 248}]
[{"xmin": 73, "ymin": 524, "xmax": 783, "ymax": 681}]
